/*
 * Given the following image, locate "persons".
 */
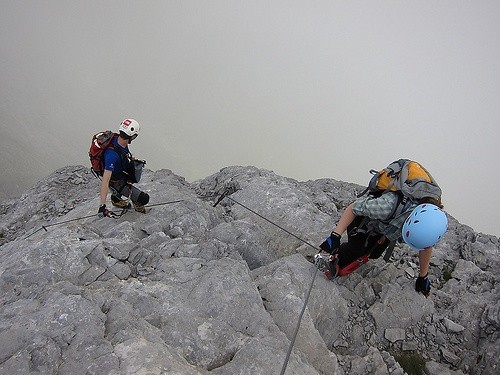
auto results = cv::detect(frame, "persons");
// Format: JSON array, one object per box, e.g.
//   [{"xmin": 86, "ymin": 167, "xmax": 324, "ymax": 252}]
[
  {"xmin": 314, "ymin": 191, "xmax": 449, "ymax": 299},
  {"xmin": 97, "ymin": 119, "xmax": 149, "ymax": 218}
]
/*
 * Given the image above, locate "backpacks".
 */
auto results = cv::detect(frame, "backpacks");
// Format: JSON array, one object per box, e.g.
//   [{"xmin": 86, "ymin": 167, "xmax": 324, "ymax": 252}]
[
  {"xmin": 88, "ymin": 130, "xmax": 129, "ymax": 177},
  {"xmin": 356, "ymin": 159, "xmax": 442, "ymax": 220}
]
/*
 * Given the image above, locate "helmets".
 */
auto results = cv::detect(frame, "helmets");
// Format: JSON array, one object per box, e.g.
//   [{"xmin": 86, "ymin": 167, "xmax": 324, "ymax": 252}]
[
  {"xmin": 401, "ymin": 203, "xmax": 448, "ymax": 250},
  {"xmin": 119, "ymin": 118, "xmax": 140, "ymax": 140}
]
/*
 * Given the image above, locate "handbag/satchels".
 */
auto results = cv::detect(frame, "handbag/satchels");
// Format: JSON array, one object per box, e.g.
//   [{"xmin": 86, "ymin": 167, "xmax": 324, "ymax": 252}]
[{"xmin": 128, "ymin": 159, "xmax": 143, "ymax": 183}]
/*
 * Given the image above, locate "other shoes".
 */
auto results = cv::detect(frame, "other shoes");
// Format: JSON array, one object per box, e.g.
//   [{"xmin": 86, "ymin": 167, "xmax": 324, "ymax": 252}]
[{"xmin": 111, "ymin": 200, "xmax": 131, "ymax": 208}]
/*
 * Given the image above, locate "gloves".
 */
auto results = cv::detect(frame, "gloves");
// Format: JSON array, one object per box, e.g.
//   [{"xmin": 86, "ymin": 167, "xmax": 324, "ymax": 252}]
[
  {"xmin": 320, "ymin": 231, "xmax": 341, "ymax": 254},
  {"xmin": 96, "ymin": 205, "xmax": 121, "ymax": 218},
  {"xmin": 416, "ymin": 272, "xmax": 431, "ymax": 297}
]
